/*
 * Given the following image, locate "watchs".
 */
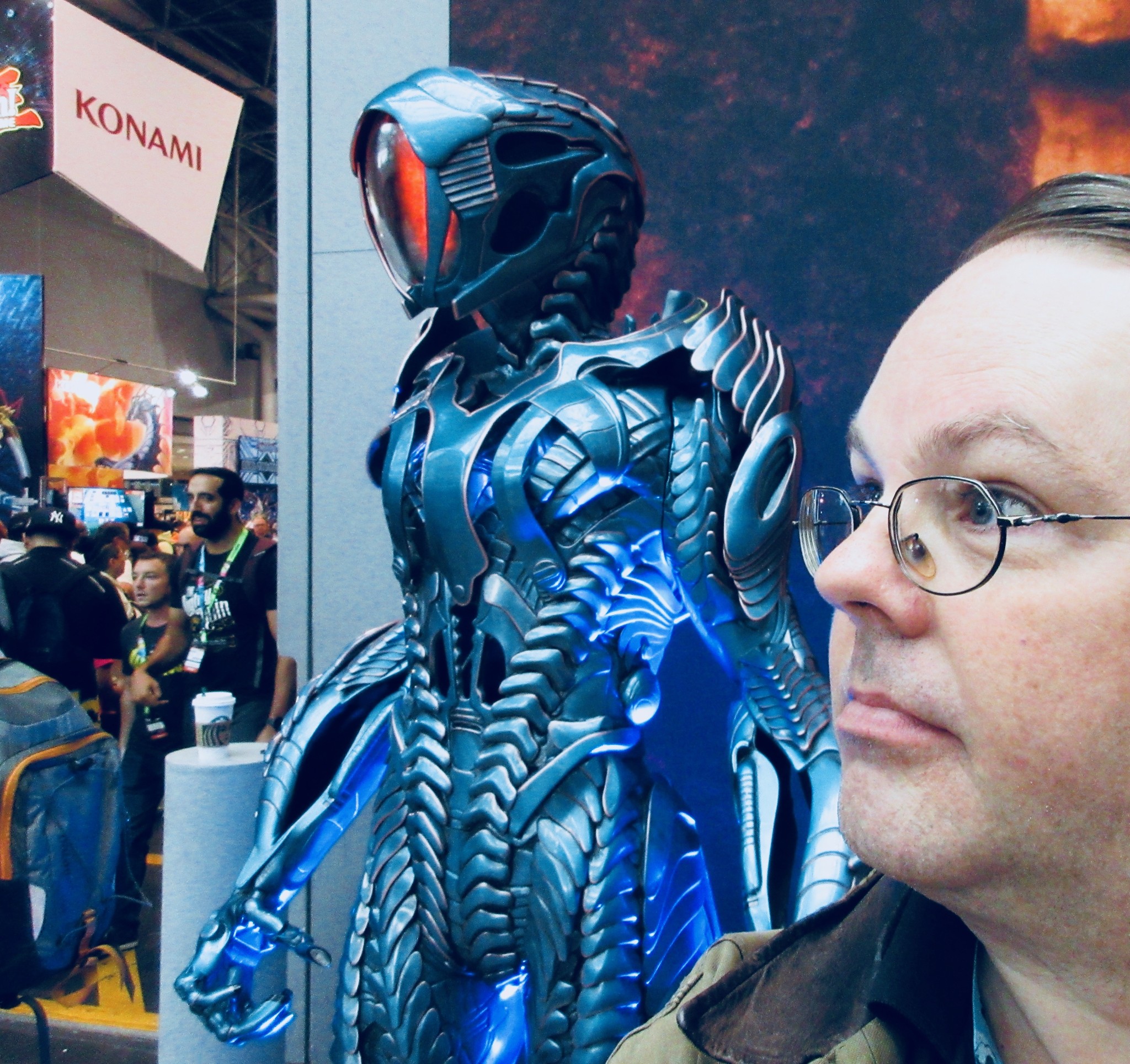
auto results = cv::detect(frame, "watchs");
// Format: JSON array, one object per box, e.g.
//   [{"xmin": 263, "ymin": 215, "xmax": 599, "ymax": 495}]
[{"xmin": 266, "ymin": 715, "xmax": 284, "ymax": 732}]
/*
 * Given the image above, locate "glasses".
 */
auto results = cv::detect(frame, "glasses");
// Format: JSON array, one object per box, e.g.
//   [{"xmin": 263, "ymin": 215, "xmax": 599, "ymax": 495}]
[
  {"xmin": 119, "ymin": 549, "xmax": 131, "ymax": 559},
  {"xmin": 792, "ymin": 474, "xmax": 1130, "ymax": 596}
]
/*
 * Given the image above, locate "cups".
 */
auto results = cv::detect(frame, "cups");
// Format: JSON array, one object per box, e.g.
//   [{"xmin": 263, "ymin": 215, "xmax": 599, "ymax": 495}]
[{"xmin": 192, "ymin": 691, "xmax": 236, "ymax": 761}]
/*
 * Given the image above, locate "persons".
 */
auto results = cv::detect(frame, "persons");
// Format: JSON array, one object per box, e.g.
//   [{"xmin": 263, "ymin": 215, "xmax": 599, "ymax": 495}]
[
  {"xmin": 0, "ymin": 465, "xmax": 296, "ymax": 955},
  {"xmin": 174, "ymin": 66, "xmax": 873, "ymax": 1064},
  {"xmin": 594, "ymin": 172, "xmax": 1130, "ymax": 1064}
]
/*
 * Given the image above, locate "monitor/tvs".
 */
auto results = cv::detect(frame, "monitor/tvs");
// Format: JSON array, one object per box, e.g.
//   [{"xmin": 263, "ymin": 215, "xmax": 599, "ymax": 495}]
[{"xmin": 66, "ymin": 486, "xmax": 144, "ymax": 537}]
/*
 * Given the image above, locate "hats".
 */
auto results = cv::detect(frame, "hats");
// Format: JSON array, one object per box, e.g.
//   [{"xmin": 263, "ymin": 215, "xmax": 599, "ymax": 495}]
[{"xmin": 25, "ymin": 506, "xmax": 76, "ymax": 540}]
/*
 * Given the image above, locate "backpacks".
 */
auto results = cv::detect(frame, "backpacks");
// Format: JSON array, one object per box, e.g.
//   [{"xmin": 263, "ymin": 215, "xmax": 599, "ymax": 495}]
[{"xmin": 1, "ymin": 647, "xmax": 153, "ymax": 1015}]
